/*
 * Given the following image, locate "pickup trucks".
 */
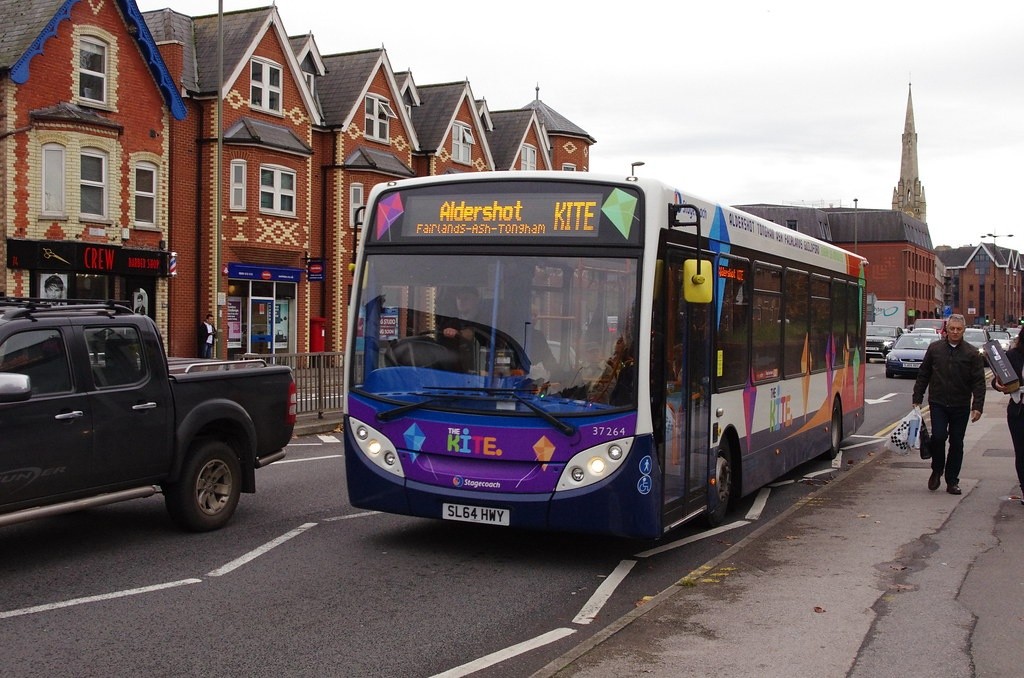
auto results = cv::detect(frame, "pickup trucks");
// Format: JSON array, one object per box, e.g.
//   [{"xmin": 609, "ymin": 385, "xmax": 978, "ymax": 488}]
[{"xmin": 0, "ymin": 294, "xmax": 298, "ymax": 535}]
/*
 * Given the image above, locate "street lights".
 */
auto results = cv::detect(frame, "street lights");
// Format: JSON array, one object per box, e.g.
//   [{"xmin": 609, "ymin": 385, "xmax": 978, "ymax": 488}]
[
  {"xmin": 521, "ymin": 320, "xmax": 532, "ymax": 353},
  {"xmin": 630, "ymin": 161, "xmax": 647, "ymax": 175},
  {"xmin": 980, "ymin": 234, "xmax": 1014, "ymax": 331},
  {"xmin": 853, "ymin": 198, "xmax": 859, "ymax": 254}
]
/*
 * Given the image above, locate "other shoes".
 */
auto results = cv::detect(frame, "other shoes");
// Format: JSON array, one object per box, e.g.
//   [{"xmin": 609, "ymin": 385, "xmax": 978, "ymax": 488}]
[
  {"xmin": 946, "ymin": 483, "xmax": 961, "ymax": 494},
  {"xmin": 1021, "ymin": 488, "xmax": 1024, "ymax": 504},
  {"xmin": 928, "ymin": 469, "xmax": 944, "ymax": 490}
]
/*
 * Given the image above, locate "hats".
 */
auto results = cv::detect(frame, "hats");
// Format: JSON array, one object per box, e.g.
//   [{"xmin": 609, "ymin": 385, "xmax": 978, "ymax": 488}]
[
  {"xmin": 585, "ymin": 341, "xmax": 600, "ymax": 352},
  {"xmin": 457, "ymin": 286, "xmax": 478, "ymax": 297}
]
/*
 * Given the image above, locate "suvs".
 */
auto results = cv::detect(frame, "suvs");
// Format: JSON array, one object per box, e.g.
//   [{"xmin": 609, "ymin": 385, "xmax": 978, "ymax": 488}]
[{"xmin": 866, "ymin": 324, "xmax": 904, "ymax": 362}]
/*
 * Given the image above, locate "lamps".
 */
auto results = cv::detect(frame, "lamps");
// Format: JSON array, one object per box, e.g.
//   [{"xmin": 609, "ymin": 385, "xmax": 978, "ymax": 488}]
[
  {"xmin": 168, "ymin": 252, "xmax": 178, "ymax": 279},
  {"xmin": 159, "ymin": 240, "xmax": 165, "ymax": 250}
]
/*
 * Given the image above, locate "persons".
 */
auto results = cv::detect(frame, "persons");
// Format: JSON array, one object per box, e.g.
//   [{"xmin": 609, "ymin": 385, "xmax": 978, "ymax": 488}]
[
  {"xmin": 436, "ymin": 286, "xmax": 499, "ymax": 378},
  {"xmin": 135, "ymin": 293, "xmax": 146, "ymax": 315},
  {"xmin": 912, "ymin": 314, "xmax": 986, "ymax": 495},
  {"xmin": 196, "ymin": 313, "xmax": 217, "ymax": 360},
  {"xmin": 991, "ymin": 319, "xmax": 1024, "ymax": 505},
  {"xmin": 41, "ymin": 275, "xmax": 64, "ymax": 306},
  {"xmin": 505, "ymin": 301, "xmax": 607, "ymax": 382}
]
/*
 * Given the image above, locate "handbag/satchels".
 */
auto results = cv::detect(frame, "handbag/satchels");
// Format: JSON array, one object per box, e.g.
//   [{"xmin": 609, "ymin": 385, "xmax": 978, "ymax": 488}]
[
  {"xmin": 884, "ymin": 409, "xmax": 914, "ymax": 456},
  {"xmin": 920, "ymin": 418, "xmax": 931, "ymax": 459},
  {"xmin": 909, "ymin": 406, "xmax": 920, "ymax": 450}
]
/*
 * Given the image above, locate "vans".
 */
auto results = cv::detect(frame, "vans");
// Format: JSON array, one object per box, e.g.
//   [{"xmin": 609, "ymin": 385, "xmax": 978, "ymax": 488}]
[{"xmin": 908, "ymin": 317, "xmax": 948, "ymax": 338}]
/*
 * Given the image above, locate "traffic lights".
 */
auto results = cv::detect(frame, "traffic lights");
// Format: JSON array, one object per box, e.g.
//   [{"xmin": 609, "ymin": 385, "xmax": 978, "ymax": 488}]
[
  {"xmin": 985, "ymin": 314, "xmax": 990, "ymax": 323},
  {"xmin": 1020, "ymin": 315, "xmax": 1024, "ymax": 324}
]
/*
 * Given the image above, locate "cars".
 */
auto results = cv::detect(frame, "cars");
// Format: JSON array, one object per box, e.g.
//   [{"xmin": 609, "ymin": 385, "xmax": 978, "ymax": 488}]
[
  {"xmin": 884, "ymin": 332, "xmax": 946, "ymax": 378},
  {"xmin": 987, "ymin": 327, "xmax": 1022, "ymax": 353},
  {"xmin": 963, "ymin": 328, "xmax": 994, "ymax": 367}
]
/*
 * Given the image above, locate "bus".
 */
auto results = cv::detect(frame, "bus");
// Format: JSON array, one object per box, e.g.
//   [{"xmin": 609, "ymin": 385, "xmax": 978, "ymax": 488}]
[{"xmin": 341, "ymin": 172, "xmax": 869, "ymax": 542}]
